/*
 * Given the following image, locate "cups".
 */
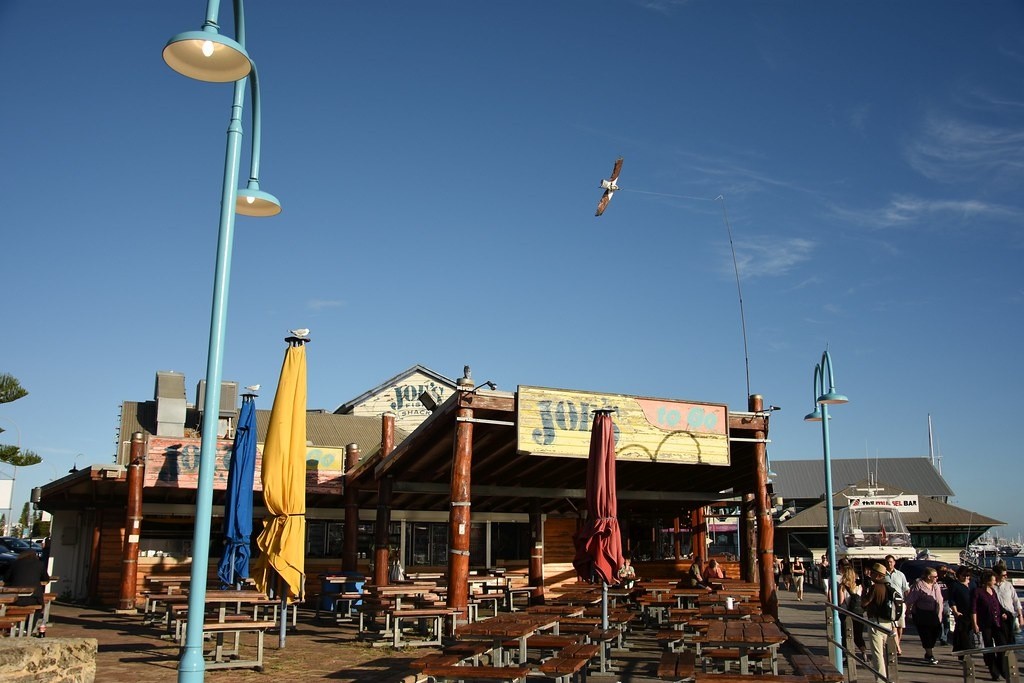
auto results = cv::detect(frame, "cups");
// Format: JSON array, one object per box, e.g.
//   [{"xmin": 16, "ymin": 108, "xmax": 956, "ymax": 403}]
[{"xmin": 726, "ymin": 597, "xmax": 735, "ymax": 609}]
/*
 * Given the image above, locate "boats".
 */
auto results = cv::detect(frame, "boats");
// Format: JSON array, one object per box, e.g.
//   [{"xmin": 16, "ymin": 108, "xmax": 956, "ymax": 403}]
[
  {"xmin": 959, "ymin": 536, "xmax": 1024, "ymax": 618},
  {"xmin": 826, "ymin": 470, "xmax": 918, "ymax": 583}
]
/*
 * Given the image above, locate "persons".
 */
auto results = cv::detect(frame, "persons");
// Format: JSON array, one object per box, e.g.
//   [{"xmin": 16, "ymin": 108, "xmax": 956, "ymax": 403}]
[
  {"xmin": 767, "ymin": 551, "xmax": 831, "ymax": 601},
  {"xmin": 688, "ymin": 556, "xmax": 723, "ymax": 593},
  {"xmin": 828, "ymin": 554, "xmax": 1024, "ymax": 683},
  {"xmin": 3, "ymin": 548, "xmax": 50, "ymax": 633},
  {"xmin": 616, "ymin": 554, "xmax": 636, "ymax": 578}
]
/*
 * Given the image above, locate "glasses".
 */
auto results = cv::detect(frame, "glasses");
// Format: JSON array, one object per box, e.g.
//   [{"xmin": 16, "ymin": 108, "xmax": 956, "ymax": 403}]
[
  {"xmin": 931, "ymin": 575, "xmax": 938, "ymax": 578},
  {"xmin": 1002, "ymin": 574, "xmax": 1007, "ymax": 576}
]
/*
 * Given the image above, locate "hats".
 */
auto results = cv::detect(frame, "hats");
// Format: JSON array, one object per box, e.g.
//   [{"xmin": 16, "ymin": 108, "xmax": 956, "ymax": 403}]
[
  {"xmin": 838, "ymin": 557, "xmax": 849, "ymax": 566},
  {"xmin": 873, "ymin": 563, "xmax": 887, "ymax": 576}
]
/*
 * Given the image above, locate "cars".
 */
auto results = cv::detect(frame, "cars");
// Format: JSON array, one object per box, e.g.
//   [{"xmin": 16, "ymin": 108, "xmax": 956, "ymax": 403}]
[{"xmin": 0, "ymin": 536, "xmax": 44, "ymax": 562}]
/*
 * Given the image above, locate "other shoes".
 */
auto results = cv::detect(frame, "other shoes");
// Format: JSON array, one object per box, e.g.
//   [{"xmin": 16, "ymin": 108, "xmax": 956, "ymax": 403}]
[
  {"xmin": 940, "ymin": 639, "xmax": 948, "ymax": 646},
  {"xmin": 843, "ymin": 659, "xmax": 848, "ymax": 668},
  {"xmin": 797, "ymin": 597, "xmax": 803, "ymax": 601}
]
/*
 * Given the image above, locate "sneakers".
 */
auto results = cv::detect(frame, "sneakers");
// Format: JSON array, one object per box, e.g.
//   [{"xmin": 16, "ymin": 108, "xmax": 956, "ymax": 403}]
[{"xmin": 924, "ymin": 653, "xmax": 939, "ymax": 665}]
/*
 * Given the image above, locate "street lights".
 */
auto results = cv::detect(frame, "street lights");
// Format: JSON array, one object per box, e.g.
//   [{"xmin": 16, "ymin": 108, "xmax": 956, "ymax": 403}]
[
  {"xmin": 160, "ymin": 0, "xmax": 285, "ymax": 683},
  {"xmin": 804, "ymin": 348, "xmax": 851, "ymax": 674},
  {"xmin": 69, "ymin": 453, "xmax": 86, "ymax": 473}
]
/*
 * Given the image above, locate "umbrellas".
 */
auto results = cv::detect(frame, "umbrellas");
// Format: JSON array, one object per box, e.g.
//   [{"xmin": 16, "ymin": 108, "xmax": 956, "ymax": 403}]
[
  {"xmin": 217, "ymin": 383, "xmax": 262, "ymax": 651},
  {"xmin": 573, "ymin": 406, "xmax": 625, "ymax": 631},
  {"xmin": 256, "ymin": 328, "xmax": 310, "ymax": 648}
]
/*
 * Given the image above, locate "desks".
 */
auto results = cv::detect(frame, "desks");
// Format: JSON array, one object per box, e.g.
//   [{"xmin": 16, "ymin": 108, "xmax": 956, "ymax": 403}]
[{"xmin": 0, "ymin": 570, "xmax": 789, "ymax": 683}]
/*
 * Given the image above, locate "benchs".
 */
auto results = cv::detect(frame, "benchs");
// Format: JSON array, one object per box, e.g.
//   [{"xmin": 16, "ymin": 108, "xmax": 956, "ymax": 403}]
[{"xmin": 2, "ymin": 577, "xmax": 846, "ymax": 683}]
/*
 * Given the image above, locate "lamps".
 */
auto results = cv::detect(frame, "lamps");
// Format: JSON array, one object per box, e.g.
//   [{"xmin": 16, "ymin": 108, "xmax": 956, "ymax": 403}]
[
  {"xmin": 417, "ymin": 388, "xmax": 440, "ymax": 413},
  {"xmin": 755, "ymin": 405, "xmax": 782, "ymax": 413},
  {"xmin": 472, "ymin": 380, "xmax": 497, "ymax": 394}
]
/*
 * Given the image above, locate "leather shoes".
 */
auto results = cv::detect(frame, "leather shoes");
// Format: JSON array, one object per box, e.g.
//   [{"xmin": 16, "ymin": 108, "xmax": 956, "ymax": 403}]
[{"xmin": 993, "ymin": 674, "xmax": 1000, "ymax": 681}]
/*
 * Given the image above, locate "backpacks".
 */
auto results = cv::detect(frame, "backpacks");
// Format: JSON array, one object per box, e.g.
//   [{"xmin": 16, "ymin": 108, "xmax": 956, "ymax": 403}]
[
  {"xmin": 879, "ymin": 583, "xmax": 903, "ymax": 621},
  {"xmin": 849, "ymin": 594, "xmax": 864, "ymax": 616}
]
